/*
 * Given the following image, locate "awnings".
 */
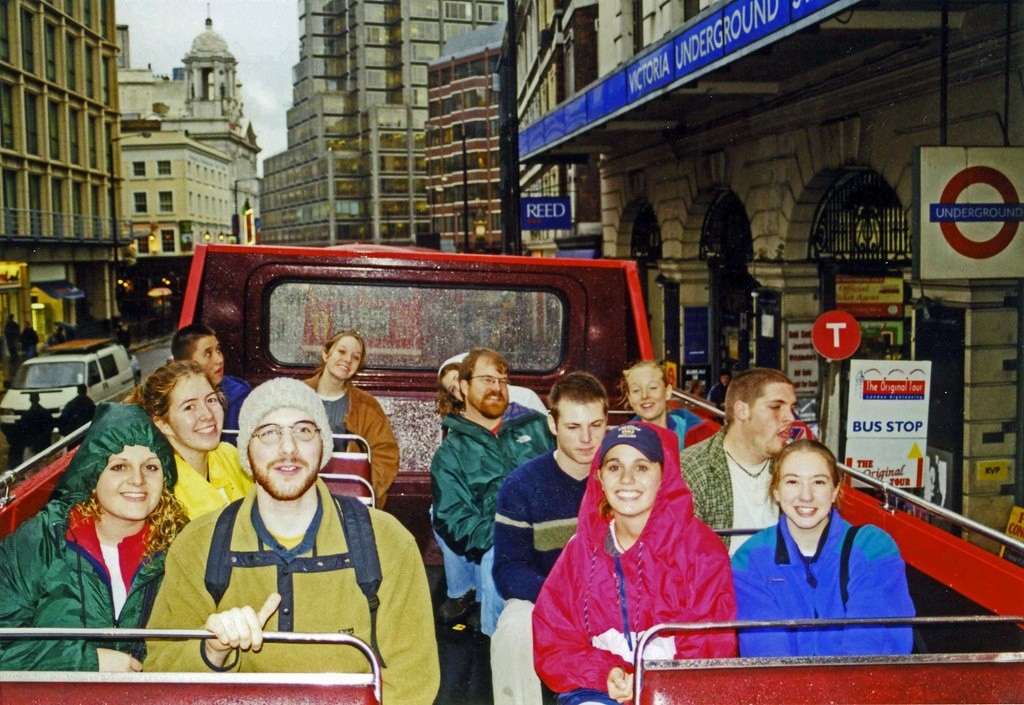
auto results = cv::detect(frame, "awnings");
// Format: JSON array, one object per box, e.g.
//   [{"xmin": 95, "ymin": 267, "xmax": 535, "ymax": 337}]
[{"xmin": 31, "ymin": 280, "xmax": 85, "ymax": 299}]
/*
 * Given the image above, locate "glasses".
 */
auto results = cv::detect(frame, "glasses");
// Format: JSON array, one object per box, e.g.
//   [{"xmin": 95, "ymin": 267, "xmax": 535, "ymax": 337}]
[
  {"xmin": 469, "ymin": 375, "xmax": 510, "ymax": 388},
  {"xmin": 252, "ymin": 423, "xmax": 321, "ymax": 444}
]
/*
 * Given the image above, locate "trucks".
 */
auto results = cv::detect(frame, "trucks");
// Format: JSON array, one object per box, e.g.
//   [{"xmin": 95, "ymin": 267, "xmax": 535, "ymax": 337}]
[{"xmin": 10, "ymin": 243, "xmax": 1024, "ymax": 705}]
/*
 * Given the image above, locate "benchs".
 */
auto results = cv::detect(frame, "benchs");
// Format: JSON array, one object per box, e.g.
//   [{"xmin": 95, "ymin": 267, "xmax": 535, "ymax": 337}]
[
  {"xmin": 608, "ymin": 409, "xmax": 1024, "ymax": 705},
  {"xmin": 0, "ymin": 433, "xmax": 383, "ymax": 705}
]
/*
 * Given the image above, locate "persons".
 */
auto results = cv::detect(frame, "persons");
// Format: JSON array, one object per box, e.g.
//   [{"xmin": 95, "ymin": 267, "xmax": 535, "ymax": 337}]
[
  {"xmin": 143, "ymin": 376, "xmax": 441, "ymax": 705},
  {"xmin": 0, "ymin": 402, "xmax": 189, "ymax": 673},
  {"xmin": 22, "ymin": 384, "xmax": 96, "ymax": 454},
  {"xmin": 533, "ymin": 422, "xmax": 736, "ymax": 705},
  {"xmin": 688, "ymin": 380, "xmax": 702, "ymax": 396},
  {"xmin": 171, "ymin": 323, "xmax": 252, "ymax": 447},
  {"xmin": 129, "ymin": 360, "xmax": 253, "ymax": 522},
  {"xmin": 117, "ymin": 320, "xmax": 131, "ymax": 348},
  {"xmin": 302, "ymin": 330, "xmax": 400, "ymax": 509},
  {"xmin": 731, "ymin": 438, "xmax": 915, "ymax": 658},
  {"xmin": 489, "ymin": 373, "xmax": 610, "ymax": 705},
  {"xmin": 20, "ymin": 324, "xmax": 39, "ymax": 358},
  {"xmin": 625, "ymin": 360, "xmax": 701, "ymax": 453},
  {"xmin": 681, "ymin": 368, "xmax": 796, "ymax": 556},
  {"xmin": 45, "ymin": 327, "xmax": 64, "ymax": 354},
  {"xmin": 707, "ymin": 368, "xmax": 732, "ymax": 411},
  {"xmin": 5, "ymin": 314, "xmax": 20, "ymax": 363},
  {"xmin": 426, "ymin": 347, "xmax": 554, "ymax": 637}
]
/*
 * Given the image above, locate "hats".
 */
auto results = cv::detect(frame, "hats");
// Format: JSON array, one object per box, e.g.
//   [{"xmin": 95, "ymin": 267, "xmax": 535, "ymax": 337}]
[
  {"xmin": 599, "ymin": 424, "xmax": 665, "ymax": 466},
  {"xmin": 236, "ymin": 377, "xmax": 334, "ymax": 477}
]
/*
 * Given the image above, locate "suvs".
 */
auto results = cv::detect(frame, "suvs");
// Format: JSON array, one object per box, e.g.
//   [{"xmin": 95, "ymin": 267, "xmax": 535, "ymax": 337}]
[{"xmin": 0, "ymin": 343, "xmax": 136, "ymax": 437}]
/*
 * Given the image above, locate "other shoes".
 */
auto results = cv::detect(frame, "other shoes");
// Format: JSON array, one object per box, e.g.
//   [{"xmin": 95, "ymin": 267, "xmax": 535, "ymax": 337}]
[{"xmin": 438, "ymin": 589, "xmax": 475, "ymax": 618}]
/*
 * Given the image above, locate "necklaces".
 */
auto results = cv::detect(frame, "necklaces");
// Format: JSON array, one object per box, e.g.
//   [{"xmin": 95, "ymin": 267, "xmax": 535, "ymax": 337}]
[
  {"xmin": 615, "ymin": 530, "xmax": 625, "ymax": 552},
  {"xmin": 726, "ymin": 451, "xmax": 769, "ymax": 479}
]
